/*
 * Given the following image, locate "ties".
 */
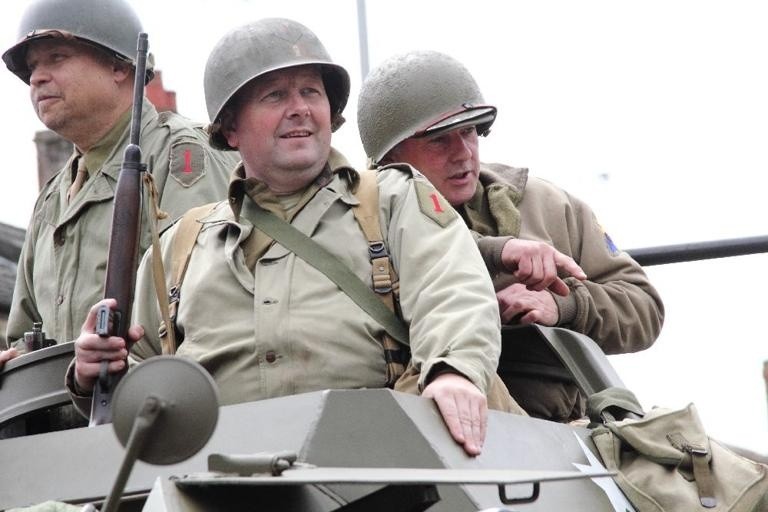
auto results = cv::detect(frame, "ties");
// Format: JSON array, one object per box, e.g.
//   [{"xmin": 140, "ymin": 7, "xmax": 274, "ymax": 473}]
[{"xmin": 68, "ymin": 156, "xmax": 88, "ymax": 204}]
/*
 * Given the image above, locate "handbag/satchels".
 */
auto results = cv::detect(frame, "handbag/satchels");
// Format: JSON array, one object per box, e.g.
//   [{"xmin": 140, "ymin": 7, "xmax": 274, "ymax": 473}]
[
  {"xmin": 394, "ymin": 359, "xmax": 529, "ymax": 416},
  {"xmin": 586, "ymin": 386, "xmax": 767, "ymax": 512}
]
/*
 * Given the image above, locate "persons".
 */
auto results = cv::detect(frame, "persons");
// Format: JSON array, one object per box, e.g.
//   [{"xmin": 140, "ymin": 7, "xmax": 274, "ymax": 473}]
[
  {"xmin": 63, "ymin": 16, "xmax": 503, "ymax": 458},
  {"xmin": 356, "ymin": 50, "xmax": 669, "ymax": 425},
  {"xmin": 1, "ymin": 1, "xmax": 243, "ymax": 428}
]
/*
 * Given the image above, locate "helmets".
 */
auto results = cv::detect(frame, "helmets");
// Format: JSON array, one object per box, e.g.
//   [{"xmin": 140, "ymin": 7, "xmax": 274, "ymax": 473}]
[
  {"xmin": 2, "ymin": 0, "xmax": 155, "ymax": 85},
  {"xmin": 358, "ymin": 50, "xmax": 497, "ymax": 169},
  {"xmin": 204, "ymin": 17, "xmax": 351, "ymax": 151}
]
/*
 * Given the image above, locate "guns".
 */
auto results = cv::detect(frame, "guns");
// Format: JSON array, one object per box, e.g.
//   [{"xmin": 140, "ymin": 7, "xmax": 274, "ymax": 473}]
[{"xmin": 88, "ymin": 31, "xmax": 148, "ymax": 424}]
